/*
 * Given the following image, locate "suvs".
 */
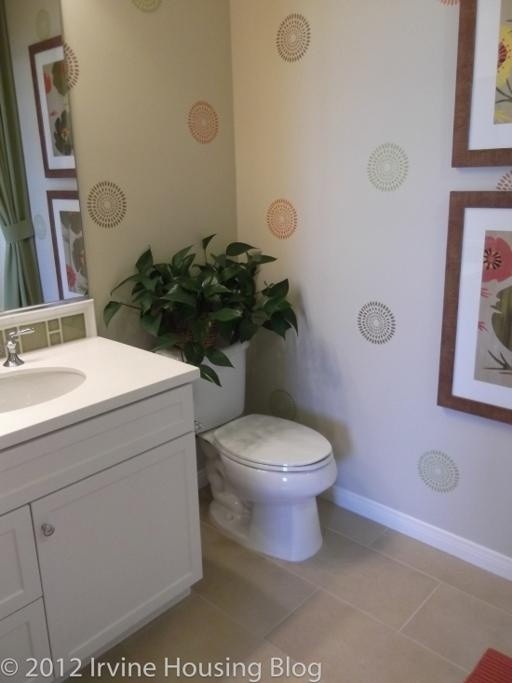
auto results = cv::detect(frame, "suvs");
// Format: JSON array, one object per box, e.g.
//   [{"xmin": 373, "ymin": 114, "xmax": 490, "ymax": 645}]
[{"xmin": 157, "ymin": 341, "xmax": 337, "ymax": 560}]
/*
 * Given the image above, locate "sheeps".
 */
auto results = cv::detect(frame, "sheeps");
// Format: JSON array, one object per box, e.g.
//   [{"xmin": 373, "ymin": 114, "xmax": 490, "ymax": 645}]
[{"xmin": 4, "ymin": 328, "xmax": 35, "ymax": 366}]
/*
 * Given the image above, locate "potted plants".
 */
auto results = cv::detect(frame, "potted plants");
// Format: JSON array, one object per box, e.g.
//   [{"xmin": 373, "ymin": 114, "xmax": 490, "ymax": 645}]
[
  {"xmin": 449, "ymin": 0, "xmax": 511, "ymax": 167},
  {"xmin": 41, "ymin": 189, "xmax": 83, "ymax": 302},
  {"xmin": 28, "ymin": 40, "xmax": 76, "ymax": 180},
  {"xmin": 437, "ymin": 189, "xmax": 512, "ymax": 430}
]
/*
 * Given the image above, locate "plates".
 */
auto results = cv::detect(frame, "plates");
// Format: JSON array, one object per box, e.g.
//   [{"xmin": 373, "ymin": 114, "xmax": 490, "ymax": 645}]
[{"xmin": 1, "ymin": 385, "xmax": 212, "ymax": 681}]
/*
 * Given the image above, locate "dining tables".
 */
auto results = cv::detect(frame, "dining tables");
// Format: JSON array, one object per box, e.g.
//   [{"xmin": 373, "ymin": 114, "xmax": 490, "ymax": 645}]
[{"xmin": 103, "ymin": 233, "xmax": 299, "ymax": 384}]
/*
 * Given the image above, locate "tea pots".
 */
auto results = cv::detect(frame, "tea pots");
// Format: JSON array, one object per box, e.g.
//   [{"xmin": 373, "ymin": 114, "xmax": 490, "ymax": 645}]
[{"xmin": 1, "ymin": 2, "xmax": 91, "ymax": 306}]
